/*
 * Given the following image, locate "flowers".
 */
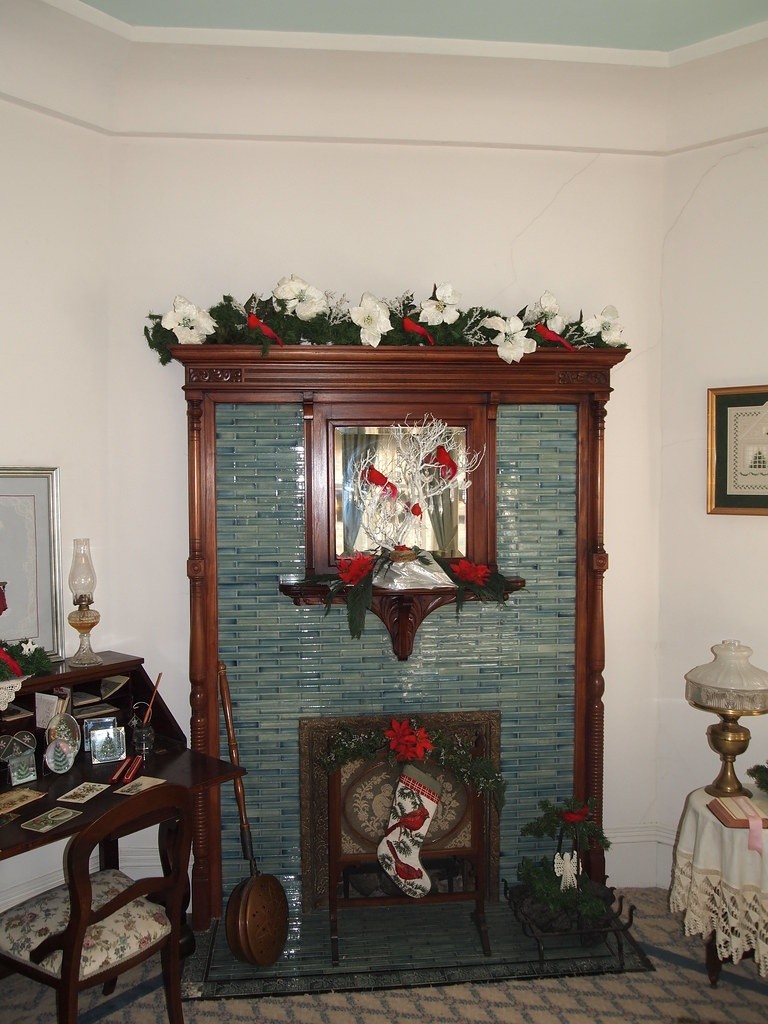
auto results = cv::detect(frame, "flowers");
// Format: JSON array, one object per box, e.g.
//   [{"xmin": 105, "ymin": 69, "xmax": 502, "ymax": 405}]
[
  {"xmin": 432, "ymin": 553, "xmax": 531, "ymax": 625},
  {"xmin": 385, "ymin": 719, "xmax": 435, "ymax": 761},
  {"xmin": 144, "ymin": 274, "xmax": 628, "ymax": 366},
  {"xmin": 296, "ymin": 551, "xmax": 373, "ymax": 640}
]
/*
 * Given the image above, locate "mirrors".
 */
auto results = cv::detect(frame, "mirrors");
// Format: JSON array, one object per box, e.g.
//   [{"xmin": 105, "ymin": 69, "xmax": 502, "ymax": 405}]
[{"xmin": 328, "ymin": 420, "xmax": 474, "ymax": 563}]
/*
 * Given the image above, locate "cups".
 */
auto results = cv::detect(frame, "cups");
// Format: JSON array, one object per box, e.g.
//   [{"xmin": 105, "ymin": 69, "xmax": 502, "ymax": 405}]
[{"xmin": 132, "ymin": 722, "xmax": 156, "ymax": 755}]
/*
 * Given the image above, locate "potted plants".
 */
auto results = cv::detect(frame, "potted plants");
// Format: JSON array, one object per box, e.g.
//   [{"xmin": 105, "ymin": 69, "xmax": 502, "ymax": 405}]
[{"xmin": 0, "ymin": 638, "xmax": 50, "ymax": 712}]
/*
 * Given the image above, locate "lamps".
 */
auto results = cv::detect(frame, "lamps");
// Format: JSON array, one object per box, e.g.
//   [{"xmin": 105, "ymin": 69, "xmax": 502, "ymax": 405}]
[
  {"xmin": 67, "ymin": 538, "xmax": 103, "ymax": 668},
  {"xmin": 685, "ymin": 639, "xmax": 768, "ymax": 798}
]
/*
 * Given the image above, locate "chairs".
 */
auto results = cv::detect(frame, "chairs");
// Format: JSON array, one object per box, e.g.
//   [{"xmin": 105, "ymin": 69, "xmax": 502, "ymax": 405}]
[{"xmin": 0, "ymin": 784, "xmax": 193, "ymax": 1024}]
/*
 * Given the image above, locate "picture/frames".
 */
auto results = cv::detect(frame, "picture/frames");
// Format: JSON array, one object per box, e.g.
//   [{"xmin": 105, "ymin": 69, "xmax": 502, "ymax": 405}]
[
  {"xmin": 0, "ymin": 466, "xmax": 66, "ymax": 663},
  {"xmin": 706, "ymin": 385, "xmax": 768, "ymax": 516},
  {"xmin": 90, "ymin": 727, "xmax": 126, "ymax": 764},
  {"xmin": 9, "ymin": 753, "xmax": 37, "ymax": 786},
  {"xmin": 84, "ymin": 717, "xmax": 117, "ymax": 751}
]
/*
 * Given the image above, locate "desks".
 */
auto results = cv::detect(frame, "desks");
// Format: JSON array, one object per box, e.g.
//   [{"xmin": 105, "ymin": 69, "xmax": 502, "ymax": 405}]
[
  {"xmin": 0, "ymin": 651, "xmax": 248, "ymax": 933},
  {"xmin": 669, "ymin": 784, "xmax": 768, "ymax": 988}
]
